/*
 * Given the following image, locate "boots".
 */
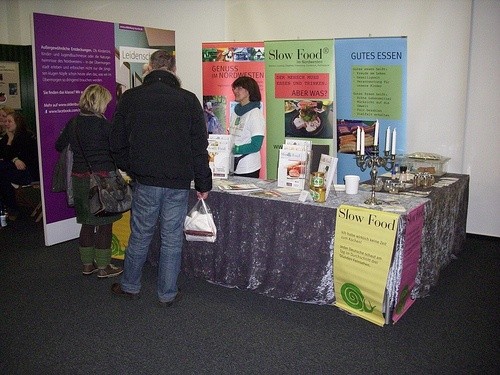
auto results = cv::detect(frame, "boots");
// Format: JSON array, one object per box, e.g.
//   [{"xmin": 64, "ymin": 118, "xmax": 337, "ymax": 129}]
[{"xmin": 14, "ymin": 192, "xmax": 27, "ymax": 217}]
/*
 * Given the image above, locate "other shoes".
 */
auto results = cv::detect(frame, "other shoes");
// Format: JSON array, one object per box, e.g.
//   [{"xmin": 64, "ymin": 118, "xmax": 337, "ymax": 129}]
[
  {"xmin": 82, "ymin": 258, "xmax": 99, "ymax": 274},
  {"xmin": 97, "ymin": 263, "xmax": 125, "ymax": 277},
  {"xmin": 111, "ymin": 282, "xmax": 140, "ymax": 301},
  {"xmin": 159, "ymin": 287, "xmax": 181, "ymax": 306}
]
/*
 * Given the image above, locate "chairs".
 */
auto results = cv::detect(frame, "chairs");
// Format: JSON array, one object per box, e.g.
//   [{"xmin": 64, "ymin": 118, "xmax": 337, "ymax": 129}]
[{"xmin": 21, "ymin": 187, "xmax": 42, "ymax": 223}]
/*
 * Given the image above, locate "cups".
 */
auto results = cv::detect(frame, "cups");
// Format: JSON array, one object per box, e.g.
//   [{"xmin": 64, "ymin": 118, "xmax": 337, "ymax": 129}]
[
  {"xmin": 375, "ymin": 178, "xmax": 382, "ymax": 191},
  {"xmin": 384, "ymin": 180, "xmax": 392, "ymax": 191},
  {"xmin": 414, "ymin": 172, "xmax": 433, "ymax": 188},
  {"xmin": 389, "ymin": 183, "xmax": 398, "ymax": 193},
  {"xmin": 0, "ymin": 215, "xmax": 7, "ymax": 226},
  {"xmin": 398, "ymin": 181, "xmax": 405, "ymax": 191},
  {"xmin": 345, "ymin": 175, "xmax": 360, "ymax": 194}
]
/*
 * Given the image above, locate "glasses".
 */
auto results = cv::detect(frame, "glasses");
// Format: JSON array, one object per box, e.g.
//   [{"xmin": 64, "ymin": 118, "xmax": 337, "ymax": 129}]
[{"xmin": 232, "ymin": 87, "xmax": 243, "ymax": 93}]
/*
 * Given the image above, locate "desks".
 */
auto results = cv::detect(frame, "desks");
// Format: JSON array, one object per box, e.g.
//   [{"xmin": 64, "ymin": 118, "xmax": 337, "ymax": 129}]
[{"xmin": 145, "ymin": 172, "xmax": 469, "ymax": 328}]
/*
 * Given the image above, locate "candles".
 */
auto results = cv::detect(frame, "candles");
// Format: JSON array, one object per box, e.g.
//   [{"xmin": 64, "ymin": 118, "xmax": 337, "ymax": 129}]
[
  {"xmin": 374, "ymin": 120, "xmax": 379, "ymax": 145},
  {"xmin": 357, "ymin": 126, "xmax": 361, "ymax": 151},
  {"xmin": 392, "ymin": 128, "xmax": 397, "ymax": 155},
  {"xmin": 386, "ymin": 126, "xmax": 391, "ymax": 151},
  {"xmin": 360, "ymin": 128, "xmax": 365, "ymax": 155}
]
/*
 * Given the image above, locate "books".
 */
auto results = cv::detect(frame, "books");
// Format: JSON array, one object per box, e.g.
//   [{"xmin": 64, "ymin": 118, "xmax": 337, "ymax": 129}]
[
  {"xmin": 318, "ymin": 153, "xmax": 338, "ymax": 200},
  {"xmin": 278, "ymin": 139, "xmax": 312, "ymax": 190}
]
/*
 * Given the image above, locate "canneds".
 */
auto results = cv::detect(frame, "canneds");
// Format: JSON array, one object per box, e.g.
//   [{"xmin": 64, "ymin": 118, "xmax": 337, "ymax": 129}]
[{"xmin": 310, "ymin": 172, "xmax": 326, "ymax": 203}]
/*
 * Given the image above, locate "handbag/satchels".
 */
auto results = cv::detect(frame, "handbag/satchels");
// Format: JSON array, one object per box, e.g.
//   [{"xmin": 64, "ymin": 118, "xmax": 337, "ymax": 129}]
[
  {"xmin": 88, "ymin": 171, "xmax": 133, "ymax": 217},
  {"xmin": 184, "ymin": 196, "xmax": 217, "ymax": 243}
]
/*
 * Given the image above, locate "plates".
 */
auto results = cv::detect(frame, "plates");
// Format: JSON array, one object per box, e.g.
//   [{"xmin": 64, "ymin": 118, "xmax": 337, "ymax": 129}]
[
  {"xmin": 285, "ymin": 101, "xmax": 296, "ymax": 113},
  {"xmin": 291, "ymin": 111, "xmax": 323, "ymax": 136}
]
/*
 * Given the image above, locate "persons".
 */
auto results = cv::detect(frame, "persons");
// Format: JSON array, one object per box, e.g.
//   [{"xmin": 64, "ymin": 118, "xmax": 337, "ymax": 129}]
[
  {"xmin": 0, "ymin": 105, "xmax": 40, "ymax": 227},
  {"xmin": 108, "ymin": 50, "xmax": 213, "ymax": 309},
  {"xmin": 228, "ymin": 76, "xmax": 265, "ymax": 179},
  {"xmin": 56, "ymin": 84, "xmax": 124, "ymax": 278}
]
